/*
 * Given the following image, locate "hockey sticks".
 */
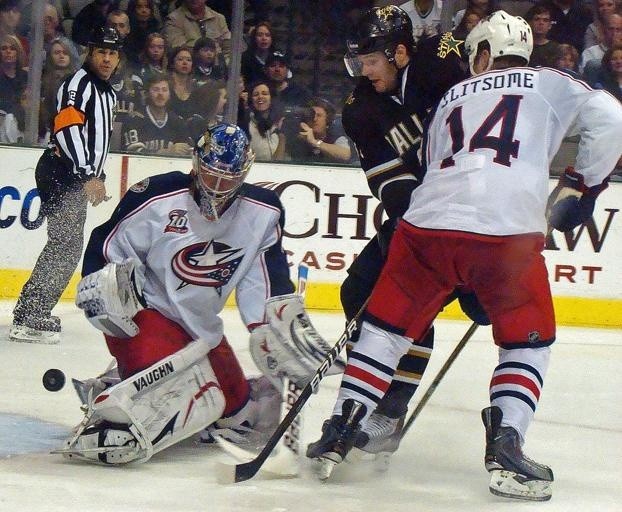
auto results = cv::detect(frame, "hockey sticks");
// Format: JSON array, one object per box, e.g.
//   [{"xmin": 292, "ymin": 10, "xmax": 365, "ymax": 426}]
[{"xmin": 213, "ymin": 261, "xmax": 373, "ymax": 485}]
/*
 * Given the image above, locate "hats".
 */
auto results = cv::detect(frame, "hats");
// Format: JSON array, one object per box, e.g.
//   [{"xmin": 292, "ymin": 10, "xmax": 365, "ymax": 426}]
[{"xmin": 265, "ymin": 50, "xmax": 290, "ymax": 66}]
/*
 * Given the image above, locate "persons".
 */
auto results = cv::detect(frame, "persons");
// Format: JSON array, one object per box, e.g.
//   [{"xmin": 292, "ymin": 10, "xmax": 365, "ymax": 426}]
[
  {"xmin": 64, "ymin": 121, "xmax": 350, "ymax": 463},
  {"xmin": 1, "ymin": 1, "xmax": 622, "ymax": 175},
  {"xmin": 11, "ymin": 25, "xmax": 126, "ymax": 332},
  {"xmin": 340, "ymin": 6, "xmax": 492, "ymax": 453},
  {"xmin": 305, "ymin": 9, "xmax": 622, "ymax": 482}
]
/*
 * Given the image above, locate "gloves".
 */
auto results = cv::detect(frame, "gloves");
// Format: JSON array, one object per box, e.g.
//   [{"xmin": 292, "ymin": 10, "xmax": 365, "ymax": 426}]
[{"xmin": 544, "ymin": 167, "xmax": 609, "ymax": 233}]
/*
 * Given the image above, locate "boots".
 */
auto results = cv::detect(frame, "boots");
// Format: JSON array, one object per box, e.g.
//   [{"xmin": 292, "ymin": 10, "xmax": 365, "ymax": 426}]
[
  {"xmin": 305, "ymin": 398, "xmax": 367, "ymax": 460},
  {"xmin": 480, "ymin": 406, "xmax": 554, "ymax": 482}
]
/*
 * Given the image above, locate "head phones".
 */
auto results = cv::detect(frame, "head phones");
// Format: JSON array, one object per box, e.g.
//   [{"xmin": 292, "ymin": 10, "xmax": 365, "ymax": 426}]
[{"xmin": 320, "ymin": 98, "xmax": 337, "ymax": 128}]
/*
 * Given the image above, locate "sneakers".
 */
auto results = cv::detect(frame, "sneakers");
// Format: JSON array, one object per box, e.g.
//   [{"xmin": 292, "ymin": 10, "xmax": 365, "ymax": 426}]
[
  {"xmin": 16, "ymin": 311, "xmax": 62, "ymax": 332},
  {"xmin": 13, "ymin": 310, "xmax": 61, "ymax": 326},
  {"xmin": 354, "ymin": 403, "xmax": 408, "ymax": 454}
]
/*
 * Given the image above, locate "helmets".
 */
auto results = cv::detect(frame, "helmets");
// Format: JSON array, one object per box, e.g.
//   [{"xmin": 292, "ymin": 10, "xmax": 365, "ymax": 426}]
[
  {"xmin": 193, "ymin": 123, "xmax": 256, "ymax": 221},
  {"xmin": 88, "ymin": 26, "xmax": 120, "ymax": 51},
  {"xmin": 344, "ymin": 5, "xmax": 417, "ymax": 78},
  {"xmin": 461, "ymin": 11, "xmax": 534, "ymax": 75}
]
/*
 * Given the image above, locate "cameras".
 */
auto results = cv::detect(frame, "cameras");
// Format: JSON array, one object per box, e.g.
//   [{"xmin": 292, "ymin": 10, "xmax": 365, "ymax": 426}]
[{"xmin": 278, "ymin": 108, "xmax": 316, "ymax": 137}]
